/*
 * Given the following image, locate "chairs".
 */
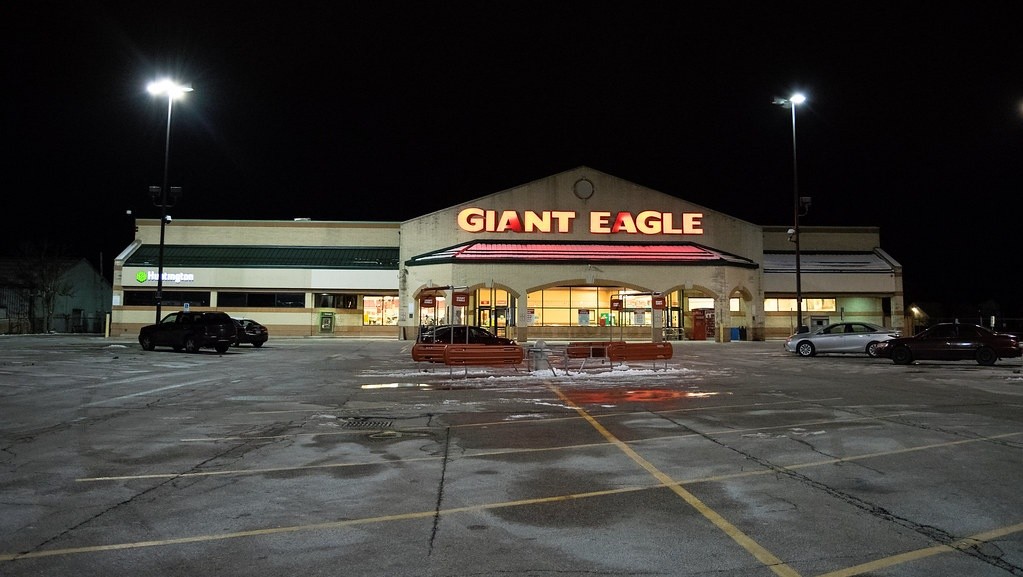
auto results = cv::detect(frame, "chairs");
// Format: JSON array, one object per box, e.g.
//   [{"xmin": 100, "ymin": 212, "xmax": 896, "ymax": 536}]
[{"xmin": 843, "ymin": 324, "xmax": 855, "ymax": 333}]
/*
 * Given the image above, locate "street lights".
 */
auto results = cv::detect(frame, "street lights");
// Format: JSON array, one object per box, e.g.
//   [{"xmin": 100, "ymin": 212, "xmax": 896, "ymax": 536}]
[
  {"xmin": 147, "ymin": 78, "xmax": 195, "ymax": 323},
  {"xmin": 772, "ymin": 92, "xmax": 806, "ymax": 332}
]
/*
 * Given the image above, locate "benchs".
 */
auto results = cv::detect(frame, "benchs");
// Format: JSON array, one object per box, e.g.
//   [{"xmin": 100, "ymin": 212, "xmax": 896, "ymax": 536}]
[
  {"xmin": 564, "ymin": 341, "xmax": 626, "ymax": 372},
  {"xmin": 444, "ymin": 345, "xmax": 525, "ymax": 379},
  {"xmin": 602, "ymin": 341, "xmax": 672, "ymax": 372},
  {"xmin": 411, "ymin": 344, "xmax": 505, "ymax": 374}
]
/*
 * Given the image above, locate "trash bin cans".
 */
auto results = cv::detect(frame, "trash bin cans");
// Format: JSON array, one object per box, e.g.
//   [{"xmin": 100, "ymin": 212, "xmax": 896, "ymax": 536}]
[
  {"xmin": 731, "ymin": 327, "xmax": 739, "ymax": 340},
  {"xmin": 740, "ymin": 326, "xmax": 747, "ymax": 340}
]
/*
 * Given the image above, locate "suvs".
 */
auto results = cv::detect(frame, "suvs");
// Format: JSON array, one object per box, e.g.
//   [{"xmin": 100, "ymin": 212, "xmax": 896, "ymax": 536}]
[{"xmin": 137, "ymin": 310, "xmax": 233, "ymax": 354}]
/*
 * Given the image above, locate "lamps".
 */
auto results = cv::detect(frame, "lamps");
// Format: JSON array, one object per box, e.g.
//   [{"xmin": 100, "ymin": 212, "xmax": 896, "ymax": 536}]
[{"xmin": 403, "ymin": 269, "xmax": 408, "ymax": 274}]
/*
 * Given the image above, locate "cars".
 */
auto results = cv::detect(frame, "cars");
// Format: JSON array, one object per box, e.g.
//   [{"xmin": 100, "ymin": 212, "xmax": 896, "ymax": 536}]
[
  {"xmin": 232, "ymin": 318, "xmax": 269, "ymax": 347},
  {"xmin": 784, "ymin": 322, "xmax": 904, "ymax": 358},
  {"xmin": 876, "ymin": 323, "xmax": 1019, "ymax": 366},
  {"xmin": 417, "ymin": 325, "xmax": 517, "ymax": 346}
]
